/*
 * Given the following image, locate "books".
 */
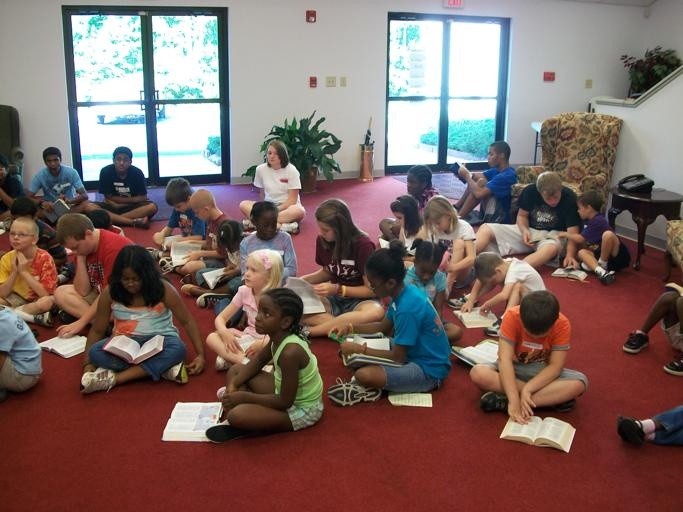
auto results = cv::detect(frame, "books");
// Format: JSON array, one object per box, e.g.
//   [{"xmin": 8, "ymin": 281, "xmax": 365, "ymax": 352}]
[
  {"xmin": 161, "ymin": 403, "xmax": 223, "ymax": 442},
  {"xmin": 665, "ymin": 282, "xmax": 683, "ymax": 296},
  {"xmin": 499, "ymin": 416, "xmax": 578, "ymax": 455}
]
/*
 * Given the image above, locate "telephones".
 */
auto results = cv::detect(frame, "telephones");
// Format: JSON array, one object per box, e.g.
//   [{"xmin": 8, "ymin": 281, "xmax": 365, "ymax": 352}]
[{"xmin": 619, "ymin": 174, "xmax": 654, "ymax": 193}]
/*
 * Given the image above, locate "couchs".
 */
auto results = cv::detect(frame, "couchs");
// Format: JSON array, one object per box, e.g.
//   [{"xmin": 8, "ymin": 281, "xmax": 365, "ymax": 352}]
[
  {"xmin": 0, "ymin": 103, "xmax": 24, "ymax": 188},
  {"xmin": 507, "ymin": 111, "xmax": 622, "ymax": 224}
]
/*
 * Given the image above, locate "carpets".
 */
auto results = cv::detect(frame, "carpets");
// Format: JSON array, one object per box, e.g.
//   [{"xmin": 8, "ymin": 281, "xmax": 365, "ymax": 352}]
[{"xmin": 390, "ymin": 171, "xmax": 482, "ymax": 201}]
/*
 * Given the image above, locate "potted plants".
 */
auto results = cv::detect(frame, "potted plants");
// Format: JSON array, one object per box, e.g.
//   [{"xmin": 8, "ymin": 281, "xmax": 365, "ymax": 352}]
[{"xmin": 239, "ymin": 109, "xmax": 343, "ymax": 194}]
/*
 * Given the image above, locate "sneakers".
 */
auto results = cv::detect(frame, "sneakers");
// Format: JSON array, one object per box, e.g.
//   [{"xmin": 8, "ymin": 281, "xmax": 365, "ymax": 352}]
[
  {"xmin": 599, "ymin": 270, "xmax": 616, "ymax": 286},
  {"xmin": 80, "ymin": 367, "xmax": 116, "ymax": 394},
  {"xmin": 206, "ymin": 419, "xmax": 231, "ymax": 442},
  {"xmin": 145, "ymin": 247, "xmax": 179, "ymax": 275},
  {"xmin": 33, "ymin": 312, "xmax": 53, "ymax": 327},
  {"xmin": 242, "ymin": 219, "xmax": 256, "ymax": 231},
  {"xmin": 280, "ymin": 222, "xmax": 300, "ymax": 234},
  {"xmin": 132, "ymin": 216, "xmax": 148, "ymax": 227},
  {"xmin": 623, "ymin": 333, "xmax": 649, "ymax": 353},
  {"xmin": 162, "ymin": 361, "xmax": 188, "ymax": 384},
  {"xmin": 617, "ymin": 416, "xmax": 645, "ymax": 448},
  {"xmin": 180, "ymin": 273, "xmax": 232, "ymax": 309},
  {"xmin": 484, "ymin": 317, "xmax": 502, "ymax": 337},
  {"xmin": 448, "ymin": 295, "xmax": 479, "ymax": 310},
  {"xmin": 480, "ymin": 391, "xmax": 508, "ymax": 413},
  {"xmin": 58, "ymin": 309, "xmax": 77, "ymax": 325},
  {"xmin": 215, "ymin": 356, "xmax": 232, "ymax": 370},
  {"xmin": 663, "ymin": 360, "xmax": 683, "ymax": 376},
  {"xmin": 579, "ymin": 261, "xmax": 594, "ymax": 272},
  {"xmin": 327, "ymin": 376, "xmax": 382, "ymax": 407}
]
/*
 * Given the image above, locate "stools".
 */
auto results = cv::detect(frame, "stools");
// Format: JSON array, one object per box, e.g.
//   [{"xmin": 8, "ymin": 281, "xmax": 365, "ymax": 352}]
[{"xmin": 660, "ymin": 218, "xmax": 683, "ymax": 283}]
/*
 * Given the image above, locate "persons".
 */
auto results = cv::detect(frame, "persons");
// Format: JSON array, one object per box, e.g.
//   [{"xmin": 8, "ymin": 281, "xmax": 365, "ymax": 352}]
[
  {"xmin": 240, "ymin": 141, "xmax": 305, "ymax": 234},
  {"xmin": 623, "ymin": 291, "xmax": 683, "ymax": 376},
  {"xmin": 300, "ymin": 164, "xmax": 588, "ymax": 425},
  {"xmin": 1, "ymin": 146, "xmax": 297, "ymax": 396},
  {"xmin": 618, "ymin": 406, "xmax": 683, "ymax": 448},
  {"xmin": 453, "ymin": 139, "xmax": 516, "ymax": 224},
  {"xmin": 205, "ymin": 288, "xmax": 323, "ymax": 443},
  {"xmin": 558, "ymin": 189, "xmax": 631, "ymax": 284}
]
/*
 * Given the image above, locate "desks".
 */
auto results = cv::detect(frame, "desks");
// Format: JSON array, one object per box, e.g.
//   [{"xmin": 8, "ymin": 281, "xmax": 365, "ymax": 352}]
[
  {"xmin": 530, "ymin": 121, "xmax": 545, "ymax": 167},
  {"xmin": 607, "ymin": 186, "xmax": 683, "ymax": 272}
]
